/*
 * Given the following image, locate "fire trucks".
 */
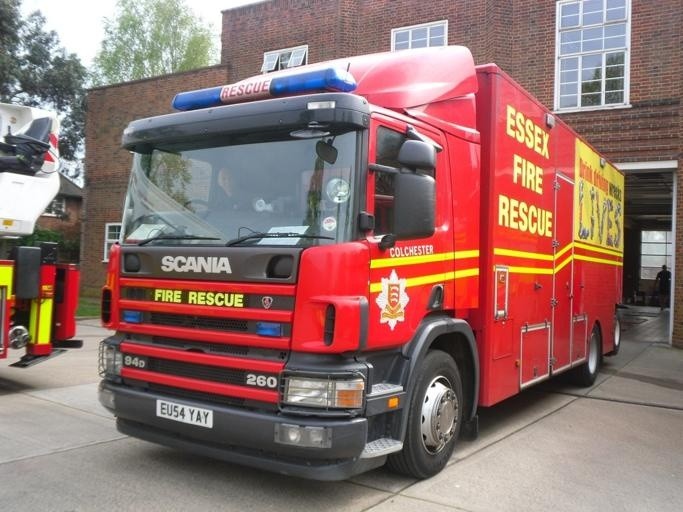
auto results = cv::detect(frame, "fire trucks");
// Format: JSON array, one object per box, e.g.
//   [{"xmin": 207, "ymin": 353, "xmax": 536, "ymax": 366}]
[
  {"xmin": 96, "ymin": 46, "xmax": 626, "ymax": 482},
  {"xmin": 0, "ymin": 101, "xmax": 84, "ymax": 369}
]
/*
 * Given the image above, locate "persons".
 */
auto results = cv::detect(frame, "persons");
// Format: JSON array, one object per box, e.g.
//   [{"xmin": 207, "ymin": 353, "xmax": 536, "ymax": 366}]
[
  {"xmin": 653, "ymin": 265, "xmax": 671, "ymax": 311},
  {"xmin": 624, "ymin": 274, "xmax": 634, "ymax": 304},
  {"xmin": 206, "ymin": 167, "xmax": 251, "ymax": 210}
]
